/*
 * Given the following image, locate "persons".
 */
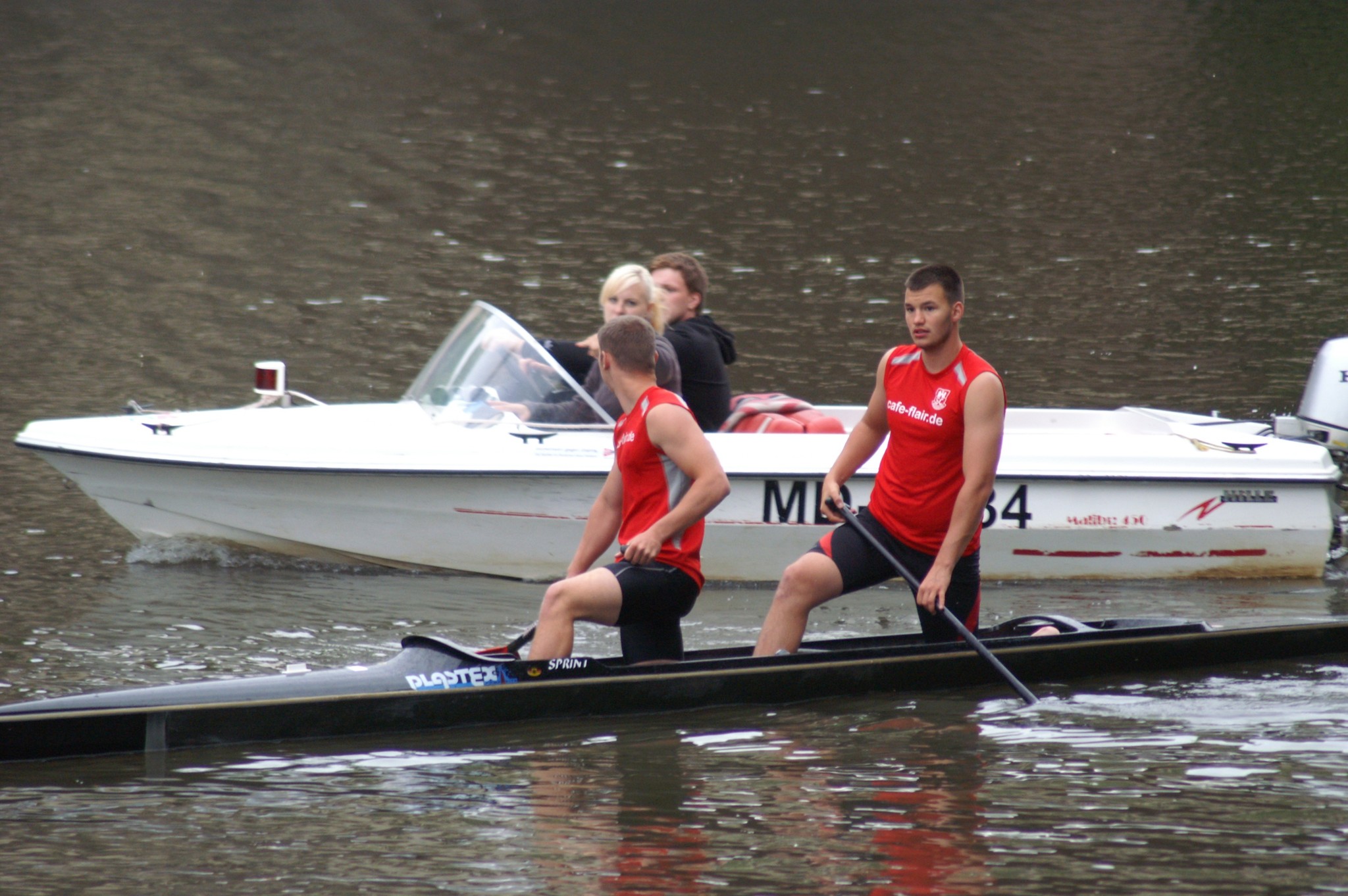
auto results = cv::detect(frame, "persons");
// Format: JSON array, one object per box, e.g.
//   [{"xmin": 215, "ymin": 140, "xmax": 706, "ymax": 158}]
[
  {"xmin": 523, "ymin": 316, "xmax": 730, "ymax": 660},
  {"xmin": 753, "ymin": 264, "xmax": 1060, "ymax": 656},
  {"xmin": 479, "ymin": 252, "xmax": 737, "ymax": 434}
]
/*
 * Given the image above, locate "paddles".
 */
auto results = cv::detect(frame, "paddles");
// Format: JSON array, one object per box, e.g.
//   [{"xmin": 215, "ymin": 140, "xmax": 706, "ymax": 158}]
[
  {"xmin": 475, "ymin": 542, "xmax": 636, "ymax": 659},
  {"xmin": 823, "ymin": 495, "xmax": 1039, "ymax": 705}
]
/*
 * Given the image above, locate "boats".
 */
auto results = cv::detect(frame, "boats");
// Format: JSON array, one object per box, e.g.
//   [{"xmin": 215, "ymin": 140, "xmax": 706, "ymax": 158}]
[
  {"xmin": 0, "ymin": 613, "xmax": 1348, "ymax": 771},
  {"xmin": 14, "ymin": 296, "xmax": 1347, "ymax": 595}
]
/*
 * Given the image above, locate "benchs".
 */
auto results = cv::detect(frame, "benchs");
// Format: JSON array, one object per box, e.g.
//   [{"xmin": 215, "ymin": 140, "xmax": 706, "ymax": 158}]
[{"xmin": 732, "ymin": 402, "xmax": 844, "ymax": 433}]
